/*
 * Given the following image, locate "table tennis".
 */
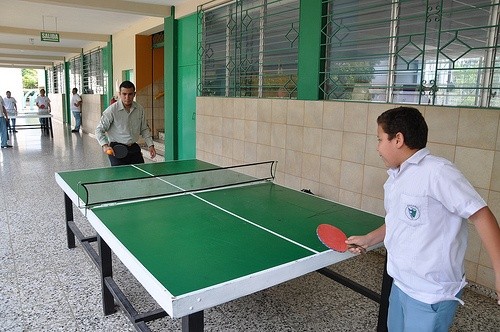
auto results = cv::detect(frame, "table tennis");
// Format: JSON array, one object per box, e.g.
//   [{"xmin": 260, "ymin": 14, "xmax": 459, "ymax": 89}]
[
  {"xmin": 40, "ymin": 103, "xmax": 45, "ymax": 111},
  {"xmin": 107, "ymin": 150, "xmax": 112, "ymax": 154}
]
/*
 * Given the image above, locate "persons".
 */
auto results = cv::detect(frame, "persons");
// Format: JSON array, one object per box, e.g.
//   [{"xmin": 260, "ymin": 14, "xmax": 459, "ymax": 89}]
[
  {"xmin": 345, "ymin": 107, "xmax": 499, "ymax": 332},
  {"xmin": 0, "ymin": 96, "xmax": 13, "ymax": 148},
  {"xmin": 72, "ymin": 88, "xmax": 82, "ymax": 132},
  {"xmin": 94, "ymin": 81, "xmax": 156, "ymax": 167},
  {"xmin": 3, "ymin": 91, "xmax": 18, "ymax": 132},
  {"xmin": 35, "ymin": 89, "xmax": 51, "ymax": 123}
]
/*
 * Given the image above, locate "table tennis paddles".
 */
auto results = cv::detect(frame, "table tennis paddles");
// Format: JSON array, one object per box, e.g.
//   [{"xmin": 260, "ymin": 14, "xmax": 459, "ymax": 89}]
[
  {"xmin": 104, "ymin": 144, "xmax": 130, "ymax": 160},
  {"xmin": 317, "ymin": 223, "xmax": 364, "ymax": 264}
]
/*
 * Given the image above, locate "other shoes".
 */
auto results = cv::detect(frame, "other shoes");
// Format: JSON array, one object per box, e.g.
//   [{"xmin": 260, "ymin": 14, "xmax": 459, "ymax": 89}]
[
  {"xmin": 13, "ymin": 130, "xmax": 18, "ymax": 132},
  {"xmin": 71, "ymin": 129, "xmax": 79, "ymax": 132},
  {"xmin": 1, "ymin": 145, "xmax": 12, "ymax": 148},
  {"xmin": 9, "ymin": 130, "xmax": 11, "ymax": 133}
]
